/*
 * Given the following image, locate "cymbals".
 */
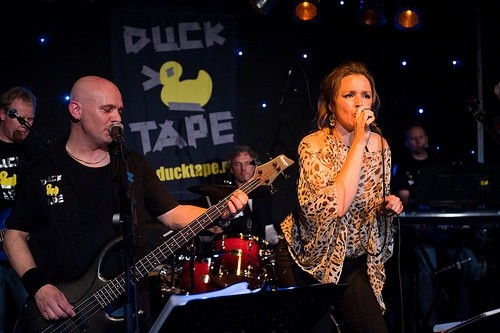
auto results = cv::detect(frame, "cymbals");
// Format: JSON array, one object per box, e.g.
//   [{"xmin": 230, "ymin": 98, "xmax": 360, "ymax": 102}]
[{"xmin": 187, "ymin": 183, "xmax": 242, "ymax": 200}]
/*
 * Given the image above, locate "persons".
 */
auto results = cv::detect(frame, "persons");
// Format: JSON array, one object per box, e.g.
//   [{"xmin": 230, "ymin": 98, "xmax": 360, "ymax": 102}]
[
  {"xmin": 395, "ymin": 125, "xmax": 486, "ymax": 333},
  {"xmin": 3, "ymin": 75, "xmax": 249, "ymax": 333},
  {"xmin": 275, "ymin": 63, "xmax": 404, "ymax": 332},
  {"xmin": 0, "ymin": 86, "xmax": 38, "ymax": 333},
  {"xmin": 202, "ymin": 146, "xmax": 279, "ymax": 247}
]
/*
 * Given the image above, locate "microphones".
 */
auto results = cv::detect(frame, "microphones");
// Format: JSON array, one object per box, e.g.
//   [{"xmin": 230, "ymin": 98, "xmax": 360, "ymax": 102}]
[
  {"xmin": 367, "ymin": 117, "xmax": 381, "ymax": 135},
  {"xmin": 109, "ymin": 122, "xmax": 124, "ymax": 142},
  {"xmin": 8, "ymin": 109, "xmax": 26, "ymax": 124}
]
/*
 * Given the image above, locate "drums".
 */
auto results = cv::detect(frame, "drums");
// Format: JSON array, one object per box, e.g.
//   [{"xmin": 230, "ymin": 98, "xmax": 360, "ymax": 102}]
[
  {"xmin": 177, "ymin": 255, "xmax": 219, "ymax": 295},
  {"xmin": 207, "ymin": 234, "xmax": 273, "ymax": 288}
]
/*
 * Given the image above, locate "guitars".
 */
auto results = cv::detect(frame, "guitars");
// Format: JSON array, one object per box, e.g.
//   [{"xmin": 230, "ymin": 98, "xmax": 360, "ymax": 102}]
[{"xmin": 12, "ymin": 152, "xmax": 295, "ymax": 333}]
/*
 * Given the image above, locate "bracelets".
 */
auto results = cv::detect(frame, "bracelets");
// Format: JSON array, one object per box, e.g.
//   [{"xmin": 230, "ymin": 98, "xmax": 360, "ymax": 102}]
[{"xmin": 19, "ymin": 266, "xmax": 50, "ymax": 295}]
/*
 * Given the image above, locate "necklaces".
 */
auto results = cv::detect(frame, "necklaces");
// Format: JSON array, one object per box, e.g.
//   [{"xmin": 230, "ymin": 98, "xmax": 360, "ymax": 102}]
[{"xmin": 66, "ymin": 143, "xmax": 109, "ymax": 163}]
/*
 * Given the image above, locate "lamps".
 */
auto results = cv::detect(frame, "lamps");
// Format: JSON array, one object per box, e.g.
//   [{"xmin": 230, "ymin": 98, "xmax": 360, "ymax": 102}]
[
  {"xmin": 392, "ymin": 2, "xmax": 424, "ymax": 32},
  {"xmin": 291, "ymin": 0, "xmax": 320, "ymax": 27}
]
